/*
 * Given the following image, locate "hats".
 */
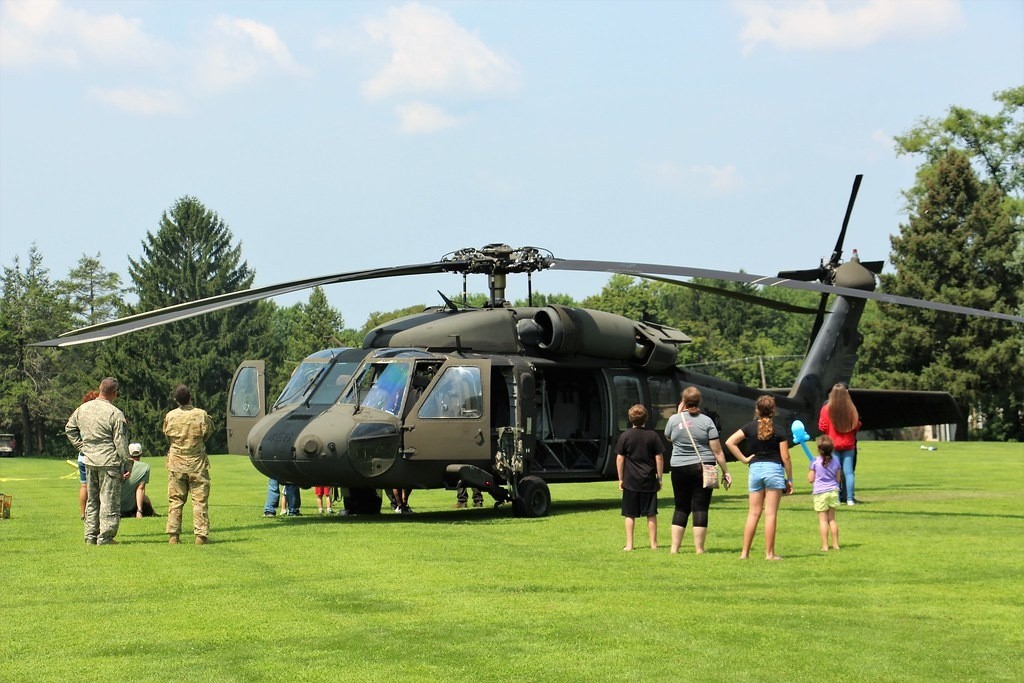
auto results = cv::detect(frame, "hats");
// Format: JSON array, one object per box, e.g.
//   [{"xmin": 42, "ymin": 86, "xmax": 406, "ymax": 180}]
[{"xmin": 128, "ymin": 442, "xmax": 143, "ymax": 456}]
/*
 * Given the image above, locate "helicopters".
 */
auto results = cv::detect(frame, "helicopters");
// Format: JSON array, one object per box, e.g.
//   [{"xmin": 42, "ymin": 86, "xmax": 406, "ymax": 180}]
[{"xmin": 26, "ymin": 174, "xmax": 1024, "ymax": 522}]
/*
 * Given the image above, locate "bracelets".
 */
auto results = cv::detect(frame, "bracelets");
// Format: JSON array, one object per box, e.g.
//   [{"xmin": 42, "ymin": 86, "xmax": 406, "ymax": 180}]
[
  {"xmin": 658, "ymin": 477, "xmax": 662, "ymax": 482},
  {"xmin": 136, "ymin": 509, "xmax": 143, "ymax": 513},
  {"xmin": 787, "ymin": 479, "xmax": 793, "ymax": 482}
]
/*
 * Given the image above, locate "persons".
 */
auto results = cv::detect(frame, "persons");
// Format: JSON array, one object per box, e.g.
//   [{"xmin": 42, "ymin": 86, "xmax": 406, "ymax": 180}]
[
  {"xmin": 78, "ymin": 391, "xmax": 159, "ymax": 519},
  {"xmin": 8, "ymin": 437, "xmax": 16, "ymax": 457},
  {"xmin": 614, "ymin": 404, "xmax": 665, "ymax": 551},
  {"xmin": 261, "ymin": 476, "xmax": 484, "ymax": 518},
  {"xmin": 725, "ymin": 395, "xmax": 794, "ymax": 559},
  {"xmin": 808, "ymin": 436, "xmax": 842, "ymax": 551},
  {"xmin": 65, "ymin": 376, "xmax": 133, "ymax": 545},
  {"xmin": 664, "ymin": 386, "xmax": 732, "ymax": 554},
  {"xmin": 819, "ymin": 382, "xmax": 862, "ymax": 506},
  {"xmin": 163, "ymin": 384, "xmax": 215, "ymax": 547}
]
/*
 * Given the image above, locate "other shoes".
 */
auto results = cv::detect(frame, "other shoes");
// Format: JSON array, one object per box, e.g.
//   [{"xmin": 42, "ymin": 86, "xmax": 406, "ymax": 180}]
[
  {"xmin": 263, "ymin": 512, "xmax": 274, "ymax": 517},
  {"xmin": 195, "ymin": 536, "xmax": 208, "ymax": 544},
  {"xmin": 81, "ymin": 517, "xmax": 84, "ymax": 520},
  {"xmin": 280, "ymin": 509, "xmax": 287, "ymax": 514},
  {"xmin": 97, "ymin": 539, "xmax": 119, "ymax": 545},
  {"xmin": 169, "ymin": 535, "xmax": 179, "ymax": 544},
  {"xmin": 327, "ymin": 509, "xmax": 335, "ymax": 514},
  {"xmin": 395, "ymin": 506, "xmax": 402, "ymax": 514},
  {"xmin": 288, "ymin": 511, "xmax": 303, "ymax": 517},
  {"xmin": 847, "ymin": 499, "xmax": 855, "ymax": 505},
  {"xmin": 87, "ymin": 539, "xmax": 96, "ymax": 544},
  {"xmin": 472, "ymin": 501, "xmax": 483, "ymax": 507},
  {"xmin": 320, "ymin": 510, "xmax": 325, "ymax": 514},
  {"xmin": 452, "ymin": 502, "xmax": 467, "ymax": 508}
]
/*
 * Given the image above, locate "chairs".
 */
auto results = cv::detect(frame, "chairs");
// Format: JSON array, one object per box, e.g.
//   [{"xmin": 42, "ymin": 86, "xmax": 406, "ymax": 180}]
[{"xmin": 536, "ymin": 400, "xmax": 604, "ymax": 470}]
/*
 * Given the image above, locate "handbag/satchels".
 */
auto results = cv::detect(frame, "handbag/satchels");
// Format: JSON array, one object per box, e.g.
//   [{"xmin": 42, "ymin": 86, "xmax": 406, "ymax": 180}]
[{"xmin": 702, "ymin": 464, "xmax": 720, "ymax": 488}]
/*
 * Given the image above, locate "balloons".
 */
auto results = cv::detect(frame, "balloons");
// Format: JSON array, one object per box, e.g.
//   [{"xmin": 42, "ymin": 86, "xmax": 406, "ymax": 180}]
[{"xmin": 791, "ymin": 419, "xmax": 814, "ymax": 462}]
